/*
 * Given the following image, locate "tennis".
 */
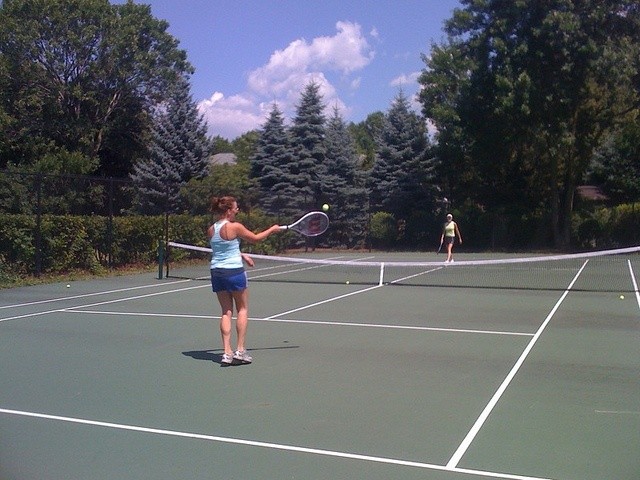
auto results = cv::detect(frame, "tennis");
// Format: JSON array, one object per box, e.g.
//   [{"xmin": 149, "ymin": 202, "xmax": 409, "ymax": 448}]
[
  {"xmin": 322, "ymin": 204, "xmax": 329, "ymax": 212},
  {"xmin": 66, "ymin": 285, "xmax": 70, "ymax": 288},
  {"xmin": 620, "ymin": 295, "xmax": 624, "ymax": 300},
  {"xmin": 345, "ymin": 280, "xmax": 350, "ymax": 284}
]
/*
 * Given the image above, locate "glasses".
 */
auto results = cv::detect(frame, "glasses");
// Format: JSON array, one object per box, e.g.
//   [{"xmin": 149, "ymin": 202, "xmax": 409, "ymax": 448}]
[{"xmin": 231, "ymin": 207, "xmax": 239, "ymax": 211}]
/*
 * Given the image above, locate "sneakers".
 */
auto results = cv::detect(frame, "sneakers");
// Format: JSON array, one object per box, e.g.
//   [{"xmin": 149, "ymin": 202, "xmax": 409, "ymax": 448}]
[
  {"xmin": 233, "ymin": 350, "xmax": 252, "ymax": 364},
  {"xmin": 221, "ymin": 353, "xmax": 242, "ymax": 365}
]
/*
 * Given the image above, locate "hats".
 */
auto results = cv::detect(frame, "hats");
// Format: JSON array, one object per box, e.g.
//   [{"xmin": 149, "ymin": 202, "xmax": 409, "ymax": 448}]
[{"xmin": 447, "ymin": 213, "xmax": 453, "ymax": 218}]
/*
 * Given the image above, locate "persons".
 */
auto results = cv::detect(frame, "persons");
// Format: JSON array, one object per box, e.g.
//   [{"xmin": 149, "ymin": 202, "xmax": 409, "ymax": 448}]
[
  {"xmin": 441, "ymin": 214, "xmax": 462, "ymax": 262},
  {"xmin": 207, "ymin": 196, "xmax": 284, "ymax": 364}
]
